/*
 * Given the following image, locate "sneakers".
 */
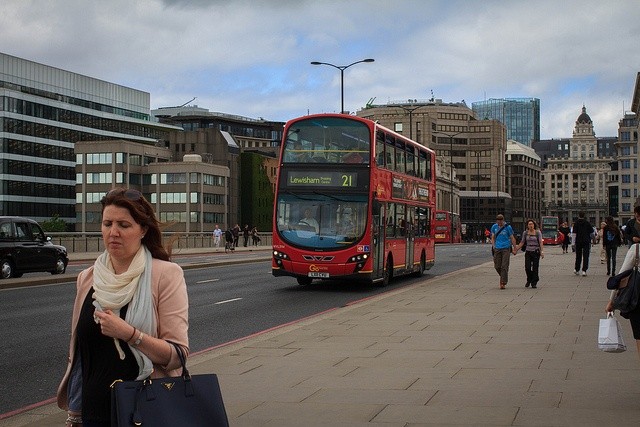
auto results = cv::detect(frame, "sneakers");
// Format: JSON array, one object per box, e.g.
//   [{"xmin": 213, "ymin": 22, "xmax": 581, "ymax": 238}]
[
  {"xmin": 582, "ymin": 271, "xmax": 587, "ymax": 276},
  {"xmin": 499, "ymin": 281, "xmax": 505, "ymax": 289},
  {"xmin": 573, "ymin": 270, "xmax": 580, "ymax": 275},
  {"xmin": 525, "ymin": 282, "xmax": 530, "ymax": 287},
  {"xmin": 531, "ymin": 284, "xmax": 537, "ymax": 288}
]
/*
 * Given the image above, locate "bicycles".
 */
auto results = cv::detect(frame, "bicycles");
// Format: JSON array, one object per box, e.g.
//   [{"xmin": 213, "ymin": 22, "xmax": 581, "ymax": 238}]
[{"xmin": 225, "ymin": 243, "xmax": 235, "ymax": 252}]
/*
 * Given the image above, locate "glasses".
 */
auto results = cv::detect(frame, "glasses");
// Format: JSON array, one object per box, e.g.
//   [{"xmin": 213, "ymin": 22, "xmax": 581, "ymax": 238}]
[{"xmin": 105, "ymin": 187, "xmax": 143, "ymax": 202}]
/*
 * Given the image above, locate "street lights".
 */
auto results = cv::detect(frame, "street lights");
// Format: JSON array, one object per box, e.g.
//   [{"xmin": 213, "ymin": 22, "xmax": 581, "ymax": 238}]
[
  {"xmin": 434, "ymin": 131, "xmax": 474, "ymax": 239},
  {"xmin": 492, "ymin": 164, "xmax": 509, "ymax": 214},
  {"xmin": 388, "ymin": 104, "xmax": 435, "ymax": 138},
  {"xmin": 464, "ymin": 149, "xmax": 491, "ymax": 221},
  {"xmin": 311, "ymin": 59, "xmax": 375, "ymax": 113}
]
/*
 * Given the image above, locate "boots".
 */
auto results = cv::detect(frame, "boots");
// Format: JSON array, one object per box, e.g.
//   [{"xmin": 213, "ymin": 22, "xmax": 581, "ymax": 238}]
[
  {"xmin": 607, "ymin": 268, "xmax": 610, "ymax": 274},
  {"xmin": 612, "ymin": 267, "xmax": 616, "ymax": 275}
]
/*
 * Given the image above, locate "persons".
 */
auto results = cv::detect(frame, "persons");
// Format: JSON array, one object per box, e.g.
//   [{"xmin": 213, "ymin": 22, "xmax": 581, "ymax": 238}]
[
  {"xmin": 298, "ymin": 207, "xmax": 319, "ymax": 234},
  {"xmin": 624, "ymin": 205, "xmax": 640, "ymax": 248},
  {"xmin": 618, "ymin": 225, "xmax": 624, "ymax": 246},
  {"xmin": 512, "ymin": 219, "xmax": 544, "ymax": 288},
  {"xmin": 605, "ymin": 241, "xmax": 640, "ymax": 358},
  {"xmin": 212, "ymin": 224, "xmax": 223, "ymax": 251},
  {"xmin": 595, "ymin": 221, "xmax": 607, "ymax": 263},
  {"xmin": 225, "ymin": 226, "xmax": 235, "ymax": 250},
  {"xmin": 603, "ymin": 215, "xmax": 621, "ymax": 276},
  {"xmin": 572, "ymin": 210, "xmax": 594, "ymax": 276},
  {"xmin": 243, "ymin": 224, "xmax": 250, "ymax": 247},
  {"xmin": 232, "ymin": 223, "xmax": 241, "ymax": 247},
  {"xmin": 557, "ymin": 221, "xmax": 576, "ymax": 253},
  {"xmin": 55, "ymin": 187, "xmax": 190, "ymax": 427},
  {"xmin": 490, "ymin": 213, "xmax": 517, "ymax": 289},
  {"xmin": 483, "ymin": 226, "xmax": 489, "ymax": 241},
  {"xmin": 621, "ymin": 222, "xmax": 628, "ymax": 246},
  {"xmin": 250, "ymin": 225, "xmax": 259, "ymax": 247},
  {"xmin": 292, "ymin": 140, "xmax": 310, "ymax": 160}
]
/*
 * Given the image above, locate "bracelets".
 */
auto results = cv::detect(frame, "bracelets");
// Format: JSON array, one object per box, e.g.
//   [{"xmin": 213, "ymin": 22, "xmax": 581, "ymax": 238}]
[{"xmin": 125, "ymin": 326, "xmax": 136, "ymax": 342}]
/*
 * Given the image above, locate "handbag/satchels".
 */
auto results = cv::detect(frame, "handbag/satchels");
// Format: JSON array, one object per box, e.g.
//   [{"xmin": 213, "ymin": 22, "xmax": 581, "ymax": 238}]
[
  {"xmin": 600, "ymin": 248, "xmax": 608, "ymax": 263},
  {"xmin": 607, "ymin": 269, "xmax": 640, "ymax": 319},
  {"xmin": 521, "ymin": 241, "xmax": 527, "ymax": 251},
  {"xmin": 598, "ymin": 318, "xmax": 627, "ymax": 353},
  {"xmin": 491, "ymin": 243, "xmax": 513, "ymax": 255},
  {"xmin": 110, "ymin": 373, "xmax": 230, "ymax": 425},
  {"xmin": 557, "ymin": 233, "xmax": 564, "ymax": 244}
]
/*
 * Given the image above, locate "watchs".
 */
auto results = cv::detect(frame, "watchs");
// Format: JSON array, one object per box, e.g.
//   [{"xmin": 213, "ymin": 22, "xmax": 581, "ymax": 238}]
[{"xmin": 130, "ymin": 331, "xmax": 144, "ymax": 347}]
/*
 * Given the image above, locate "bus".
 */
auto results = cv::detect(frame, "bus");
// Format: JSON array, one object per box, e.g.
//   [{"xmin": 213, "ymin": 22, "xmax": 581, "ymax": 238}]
[
  {"xmin": 542, "ymin": 216, "xmax": 559, "ymax": 244},
  {"xmin": 271, "ymin": 114, "xmax": 435, "ymax": 286},
  {"xmin": 435, "ymin": 210, "xmax": 461, "ymax": 243}
]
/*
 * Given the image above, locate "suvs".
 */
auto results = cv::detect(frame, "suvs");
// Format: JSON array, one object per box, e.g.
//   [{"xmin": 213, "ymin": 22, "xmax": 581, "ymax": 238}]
[{"xmin": 0, "ymin": 216, "xmax": 69, "ymax": 278}]
